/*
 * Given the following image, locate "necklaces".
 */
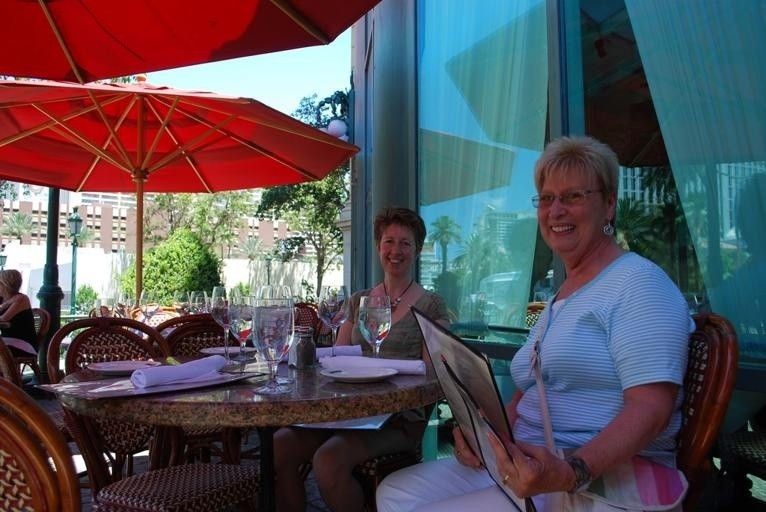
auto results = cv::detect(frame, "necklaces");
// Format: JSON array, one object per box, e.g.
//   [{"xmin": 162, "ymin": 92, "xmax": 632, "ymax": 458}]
[{"xmin": 378, "ymin": 277, "xmax": 413, "ymax": 313}]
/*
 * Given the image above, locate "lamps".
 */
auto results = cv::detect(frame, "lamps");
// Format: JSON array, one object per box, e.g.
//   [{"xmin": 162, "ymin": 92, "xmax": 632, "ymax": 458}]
[{"xmin": 313, "ymin": 90, "xmax": 349, "ymax": 145}]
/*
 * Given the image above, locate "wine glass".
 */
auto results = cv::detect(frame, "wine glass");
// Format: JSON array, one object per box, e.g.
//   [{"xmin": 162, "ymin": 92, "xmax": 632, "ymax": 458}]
[
  {"xmin": 94, "ymin": 285, "xmax": 294, "ymax": 398},
  {"xmin": 319, "ymin": 285, "xmax": 350, "ymax": 356},
  {"xmin": 357, "ymin": 295, "xmax": 391, "ymax": 357}
]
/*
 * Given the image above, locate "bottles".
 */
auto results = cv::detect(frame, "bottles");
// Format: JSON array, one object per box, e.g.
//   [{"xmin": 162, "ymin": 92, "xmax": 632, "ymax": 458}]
[{"xmin": 289, "ymin": 326, "xmax": 317, "ymax": 367}]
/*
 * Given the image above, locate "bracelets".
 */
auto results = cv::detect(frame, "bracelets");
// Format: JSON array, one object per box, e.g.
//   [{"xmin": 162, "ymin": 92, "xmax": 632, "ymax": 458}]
[{"xmin": 565, "ymin": 457, "xmax": 592, "ymax": 496}]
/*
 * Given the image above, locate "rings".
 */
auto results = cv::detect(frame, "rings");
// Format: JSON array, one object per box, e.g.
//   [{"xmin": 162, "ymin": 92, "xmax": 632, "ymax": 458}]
[
  {"xmin": 455, "ymin": 449, "xmax": 461, "ymax": 455},
  {"xmin": 501, "ymin": 473, "xmax": 513, "ymax": 484}
]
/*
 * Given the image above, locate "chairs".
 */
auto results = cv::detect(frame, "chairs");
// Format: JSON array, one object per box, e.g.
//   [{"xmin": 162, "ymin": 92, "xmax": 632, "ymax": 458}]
[
  {"xmin": 146, "ymin": 312, "xmax": 226, "ymax": 347},
  {"xmin": 9, "ymin": 307, "xmax": 51, "ymax": 398},
  {"xmin": 279, "ymin": 302, "xmax": 323, "ymax": 337},
  {"xmin": 88, "ymin": 306, "xmax": 109, "ymax": 319},
  {"xmin": 148, "ymin": 315, "xmax": 252, "ymax": 466},
  {"xmin": 0, "ymin": 363, "xmax": 80, "ymax": 512},
  {"xmin": 673, "ymin": 308, "xmax": 741, "ymax": 511},
  {"xmin": 44, "ymin": 319, "xmax": 261, "ymax": 507}
]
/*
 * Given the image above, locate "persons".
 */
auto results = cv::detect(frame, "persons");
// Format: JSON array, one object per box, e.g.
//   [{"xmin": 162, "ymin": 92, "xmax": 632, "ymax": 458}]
[
  {"xmin": 273, "ymin": 208, "xmax": 450, "ymax": 511},
  {"xmin": 377, "ymin": 138, "xmax": 695, "ymax": 512},
  {"xmin": 0, "ymin": 270, "xmax": 38, "ymax": 361}
]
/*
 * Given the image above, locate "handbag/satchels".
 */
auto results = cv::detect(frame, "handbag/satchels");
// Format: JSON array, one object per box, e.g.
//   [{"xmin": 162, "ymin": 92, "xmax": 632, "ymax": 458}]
[{"xmin": 543, "ymin": 447, "xmax": 688, "ymax": 511}]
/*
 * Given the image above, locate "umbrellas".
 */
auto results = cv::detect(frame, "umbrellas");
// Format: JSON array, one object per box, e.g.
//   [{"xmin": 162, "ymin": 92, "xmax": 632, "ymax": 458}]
[
  {"xmin": 0, "ymin": 86, "xmax": 360, "ymax": 328},
  {"xmin": 0, "ymin": 0, "xmax": 379, "ymax": 84}
]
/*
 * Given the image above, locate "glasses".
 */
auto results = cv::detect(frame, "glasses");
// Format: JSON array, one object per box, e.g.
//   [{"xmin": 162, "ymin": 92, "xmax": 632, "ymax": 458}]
[{"xmin": 531, "ymin": 188, "xmax": 602, "ymax": 207}]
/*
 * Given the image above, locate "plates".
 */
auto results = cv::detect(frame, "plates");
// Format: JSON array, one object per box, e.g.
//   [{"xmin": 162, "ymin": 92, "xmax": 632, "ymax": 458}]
[
  {"xmin": 317, "ymin": 367, "xmax": 397, "ymax": 383},
  {"xmin": 89, "ymin": 358, "xmax": 160, "ymax": 376}
]
